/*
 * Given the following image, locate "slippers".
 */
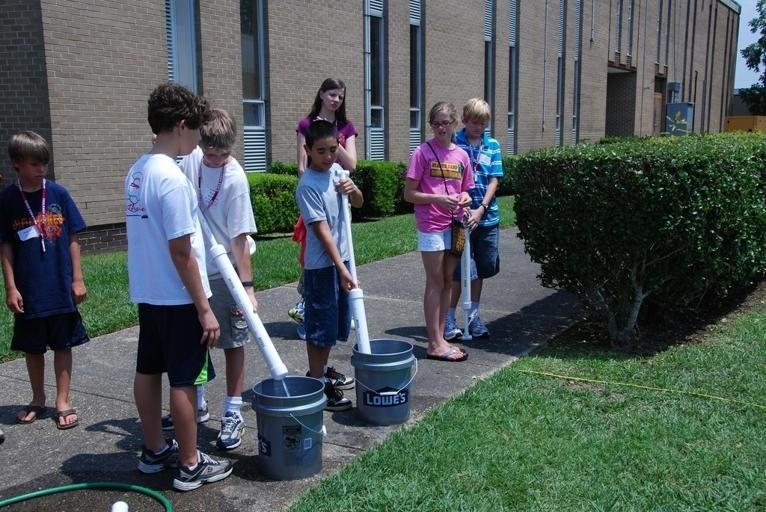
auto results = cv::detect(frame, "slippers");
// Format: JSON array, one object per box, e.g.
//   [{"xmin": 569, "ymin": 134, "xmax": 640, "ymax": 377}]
[
  {"xmin": 55, "ymin": 408, "xmax": 78, "ymax": 429},
  {"xmin": 16, "ymin": 405, "xmax": 48, "ymax": 424},
  {"xmin": 426, "ymin": 345, "xmax": 468, "ymax": 362}
]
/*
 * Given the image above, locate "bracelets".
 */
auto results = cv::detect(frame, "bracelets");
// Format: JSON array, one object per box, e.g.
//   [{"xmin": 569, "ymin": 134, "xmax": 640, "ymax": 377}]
[{"xmin": 240, "ymin": 281, "xmax": 257, "ymax": 288}]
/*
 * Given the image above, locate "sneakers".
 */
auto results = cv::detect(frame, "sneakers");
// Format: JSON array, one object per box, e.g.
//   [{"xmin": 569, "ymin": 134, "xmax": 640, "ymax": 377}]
[
  {"xmin": 216, "ymin": 411, "xmax": 246, "ymax": 450},
  {"xmin": 173, "ymin": 449, "xmax": 233, "ymax": 491},
  {"xmin": 306, "ymin": 369, "xmax": 355, "ymax": 390},
  {"xmin": 138, "ymin": 438, "xmax": 180, "ymax": 474},
  {"xmin": 445, "ymin": 315, "xmax": 463, "ymax": 341},
  {"xmin": 323, "ymin": 382, "xmax": 352, "ymax": 411},
  {"xmin": 469, "ymin": 314, "xmax": 491, "ymax": 338},
  {"xmin": 162, "ymin": 401, "xmax": 209, "ymax": 431},
  {"xmin": 297, "ymin": 326, "xmax": 307, "ymax": 340}
]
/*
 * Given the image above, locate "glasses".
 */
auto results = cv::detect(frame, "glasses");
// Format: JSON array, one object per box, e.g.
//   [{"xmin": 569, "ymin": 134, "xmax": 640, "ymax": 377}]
[{"xmin": 430, "ymin": 119, "xmax": 454, "ymax": 128}]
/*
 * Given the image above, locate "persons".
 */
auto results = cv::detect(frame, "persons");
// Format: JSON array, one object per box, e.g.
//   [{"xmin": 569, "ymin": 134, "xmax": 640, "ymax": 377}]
[
  {"xmin": 295, "ymin": 120, "xmax": 364, "ymax": 411},
  {"xmin": 288, "ymin": 215, "xmax": 309, "ymax": 341},
  {"xmin": 148, "ymin": 106, "xmax": 260, "ymax": 450},
  {"xmin": 404, "ymin": 101, "xmax": 475, "ymax": 363},
  {"xmin": 444, "ymin": 97, "xmax": 504, "ymax": 342},
  {"xmin": 1, "ymin": 130, "xmax": 88, "ymax": 430},
  {"xmin": 294, "ymin": 77, "xmax": 358, "ymax": 331},
  {"xmin": 130, "ymin": 80, "xmax": 233, "ymax": 493}
]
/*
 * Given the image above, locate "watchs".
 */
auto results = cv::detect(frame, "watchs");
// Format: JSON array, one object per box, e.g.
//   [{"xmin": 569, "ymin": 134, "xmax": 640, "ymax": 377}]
[{"xmin": 480, "ymin": 202, "xmax": 489, "ymax": 212}]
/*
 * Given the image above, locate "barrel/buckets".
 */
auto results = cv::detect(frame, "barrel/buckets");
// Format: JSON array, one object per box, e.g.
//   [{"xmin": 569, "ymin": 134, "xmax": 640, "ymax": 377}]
[
  {"xmin": 251, "ymin": 375, "xmax": 328, "ymax": 482},
  {"xmin": 350, "ymin": 338, "xmax": 419, "ymax": 426}
]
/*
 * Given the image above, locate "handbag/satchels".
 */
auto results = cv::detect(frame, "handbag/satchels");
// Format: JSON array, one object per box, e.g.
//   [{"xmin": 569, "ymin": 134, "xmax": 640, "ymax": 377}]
[
  {"xmin": 288, "ymin": 296, "xmax": 306, "ymax": 323},
  {"xmin": 449, "ymin": 217, "xmax": 464, "ymax": 256}
]
